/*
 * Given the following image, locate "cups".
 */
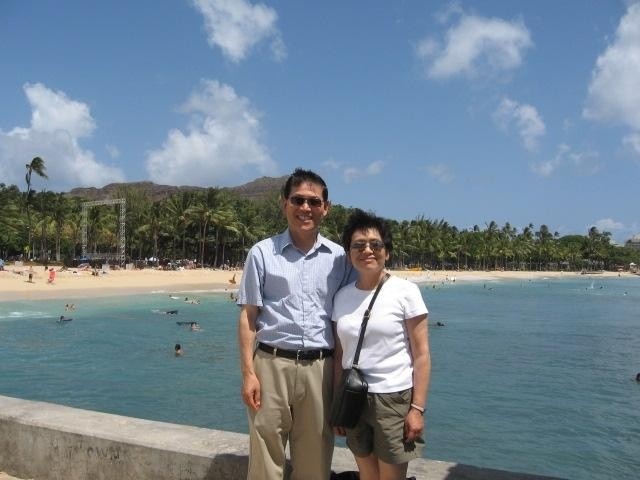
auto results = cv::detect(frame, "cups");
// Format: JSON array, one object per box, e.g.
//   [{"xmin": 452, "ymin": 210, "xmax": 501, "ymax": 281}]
[
  {"xmin": 288, "ymin": 197, "xmax": 325, "ymax": 207},
  {"xmin": 350, "ymin": 241, "xmax": 384, "ymax": 249}
]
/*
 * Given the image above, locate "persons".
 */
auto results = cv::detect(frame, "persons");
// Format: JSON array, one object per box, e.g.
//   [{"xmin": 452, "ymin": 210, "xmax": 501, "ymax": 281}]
[
  {"xmin": 60, "ymin": 315, "xmax": 65, "ymax": 321},
  {"xmin": 0, "ymin": 250, "xmax": 236, "ymax": 288},
  {"xmin": 191, "ymin": 323, "xmax": 198, "ymax": 332},
  {"xmin": 174, "ymin": 343, "xmax": 184, "ymax": 356},
  {"xmin": 635, "ymin": 373, "xmax": 640, "ymax": 381},
  {"xmin": 326, "ymin": 209, "xmax": 430, "ymax": 480},
  {"xmin": 436, "ymin": 321, "xmax": 446, "ymax": 326},
  {"xmin": 238, "ymin": 166, "xmax": 359, "ymax": 479}
]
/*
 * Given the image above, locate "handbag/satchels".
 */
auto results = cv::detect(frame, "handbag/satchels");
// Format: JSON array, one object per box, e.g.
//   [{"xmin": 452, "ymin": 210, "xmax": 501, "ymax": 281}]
[{"xmin": 410, "ymin": 403, "xmax": 427, "ymax": 414}]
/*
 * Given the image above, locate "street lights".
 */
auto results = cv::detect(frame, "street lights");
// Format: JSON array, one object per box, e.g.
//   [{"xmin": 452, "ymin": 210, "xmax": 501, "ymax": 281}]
[{"xmin": 328, "ymin": 368, "xmax": 368, "ymax": 429}]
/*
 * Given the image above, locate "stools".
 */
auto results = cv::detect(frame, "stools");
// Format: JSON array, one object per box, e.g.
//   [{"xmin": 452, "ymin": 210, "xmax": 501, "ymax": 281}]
[{"xmin": 258, "ymin": 343, "xmax": 335, "ymax": 360}]
